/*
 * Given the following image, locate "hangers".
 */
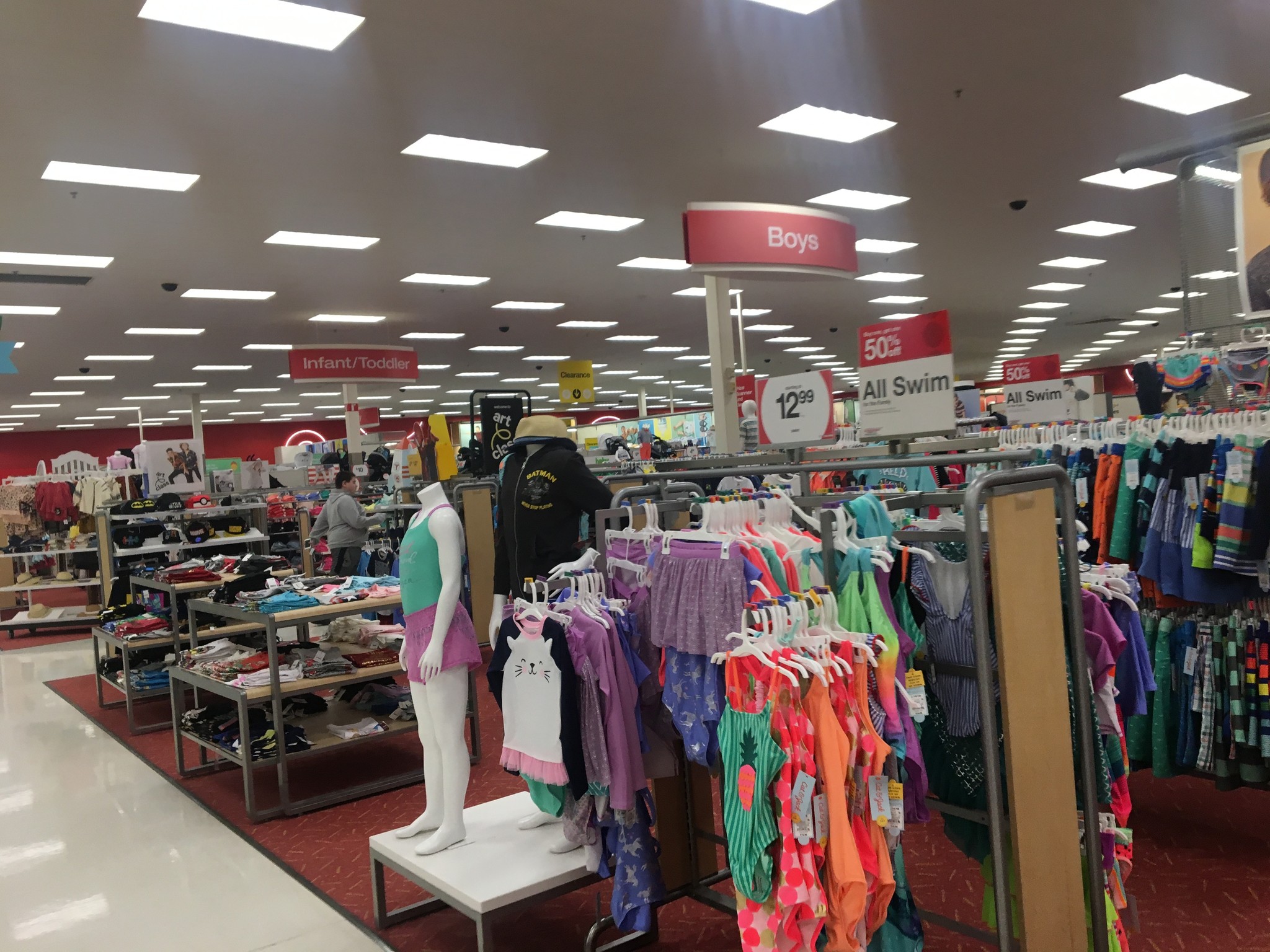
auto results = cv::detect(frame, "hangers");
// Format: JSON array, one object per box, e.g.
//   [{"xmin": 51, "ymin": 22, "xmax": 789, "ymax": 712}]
[
  {"xmin": 1158, "ymin": 326, "xmax": 1270, "ymax": 359},
  {"xmin": 513, "ymin": 487, "xmax": 989, "ymax": 689},
  {"xmin": 620, "ymin": 427, "xmax": 961, "ymax": 482},
  {"xmin": 1, "ymin": 475, "xmax": 116, "ymax": 486},
  {"xmin": 968, "ymin": 407, "xmax": 1270, "ymax": 466}
]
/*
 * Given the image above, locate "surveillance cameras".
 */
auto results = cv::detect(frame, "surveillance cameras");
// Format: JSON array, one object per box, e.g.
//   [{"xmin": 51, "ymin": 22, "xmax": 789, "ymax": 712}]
[
  {"xmin": 535, "ymin": 366, "xmax": 543, "ymax": 370},
  {"xmin": 829, "ymin": 328, "xmax": 838, "ymax": 332},
  {"xmin": 608, "ymin": 400, "xmax": 623, "ymax": 409},
  {"xmin": 850, "ymin": 385, "xmax": 854, "ymax": 387},
  {"xmin": 1170, "ymin": 287, "xmax": 1181, "ymax": 293},
  {"xmin": 399, "ymin": 389, "xmax": 406, "ymax": 392},
  {"xmin": 1008, "ymin": 200, "xmax": 1028, "ymax": 211},
  {"xmin": 764, "ymin": 359, "xmax": 771, "ymax": 362},
  {"xmin": 78, "ymin": 368, "xmax": 91, "ymax": 374},
  {"xmin": 499, "ymin": 325, "xmax": 509, "ymax": 332},
  {"xmin": 1152, "ymin": 322, "xmax": 1160, "ymax": 327},
  {"xmin": 160, "ymin": 283, "xmax": 178, "ymax": 292}
]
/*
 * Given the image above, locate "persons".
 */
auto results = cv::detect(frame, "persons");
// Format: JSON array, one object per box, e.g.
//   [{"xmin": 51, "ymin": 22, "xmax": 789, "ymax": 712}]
[
  {"xmin": 245, "ymin": 460, "xmax": 268, "ymax": 490},
  {"xmin": 621, "ymin": 426, "xmax": 639, "ymax": 445},
  {"xmin": 106, "ymin": 450, "xmax": 132, "ymax": 472},
  {"xmin": 474, "ymin": 426, "xmax": 482, "ymax": 442},
  {"xmin": 308, "ymin": 470, "xmax": 388, "ymax": 578},
  {"xmin": 394, "ymin": 481, "xmax": 482, "ymax": 856},
  {"xmin": 637, "ymin": 424, "xmax": 654, "ymax": 462},
  {"xmin": 738, "ymin": 399, "xmax": 759, "ymax": 454},
  {"xmin": 954, "ymin": 393, "xmax": 968, "ymax": 434},
  {"xmin": 673, "ymin": 420, "xmax": 695, "ymax": 438},
  {"xmin": 1131, "ymin": 357, "xmax": 1167, "ymax": 419},
  {"xmin": 132, "ymin": 440, "xmax": 148, "ymax": 468},
  {"xmin": 706, "ymin": 426, "xmax": 716, "ymax": 455},
  {"xmin": 487, "ymin": 415, "xmax": 628, "ymax": 652},
  {"xmin": 165, "ymin": 443, "xmax": 202, "ymax": 484},
  {"xmin": 1062, "ymin": 380, "xmax": 1090, "ymax": 424},
  {"xmin": 615, "ymin": 446, "xmax": 631, "ymax": 463}
]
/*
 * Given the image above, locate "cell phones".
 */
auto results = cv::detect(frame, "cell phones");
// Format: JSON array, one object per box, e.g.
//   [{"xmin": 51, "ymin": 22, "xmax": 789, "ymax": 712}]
[{"xmin": 304, "ymin": 538, "xmax": 311, "ymax": 549}]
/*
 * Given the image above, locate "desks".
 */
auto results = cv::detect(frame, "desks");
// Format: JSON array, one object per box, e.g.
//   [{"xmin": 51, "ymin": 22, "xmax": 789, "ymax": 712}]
[{"xmin": 369, "ymin": 790, "xmax": 616, "ymax": 952}]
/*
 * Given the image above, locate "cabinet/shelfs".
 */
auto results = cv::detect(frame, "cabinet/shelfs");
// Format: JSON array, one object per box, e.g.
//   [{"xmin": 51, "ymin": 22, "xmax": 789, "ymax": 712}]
[{"xmin": 0, "ymin": 480, "xmax": 491, "ymax": 824}]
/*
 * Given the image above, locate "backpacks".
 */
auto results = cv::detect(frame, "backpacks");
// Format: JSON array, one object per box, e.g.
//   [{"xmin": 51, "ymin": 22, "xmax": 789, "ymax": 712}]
[
  {"xmin": 651, "ymin": 434, "xmax": 677, "ymax": 458},
  {"xmin": 605, "ymin": 436, "xmax": 630, "ymax": 454}
]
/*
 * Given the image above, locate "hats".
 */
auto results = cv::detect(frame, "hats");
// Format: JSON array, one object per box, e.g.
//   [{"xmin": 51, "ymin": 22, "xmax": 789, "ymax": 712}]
[
  {"xmin": 15, "ymin": 572, "xmax": 43, "ymax": 588},
  {"xmin": 76, "ymin": 603, "xmax": 102, "ymax": 618},
  {"xmin": 223, "ymin": 517, "xmax": 251, "ymax": 537},
  {"xmin": 220, "ymin": 493, "xmax": 249, "ymax": 507},
  {"xmin": 117, "ymin": 532, "xmax": 146, "ymax": 548},
  {"xmin": 156, "ymin": 493, "xmax": 184, "ymax": 512},
  {"xmin": 110, "ymin": 503, "xmax": 125, "ymax": 515},
  {"xmin": 142, "ymin": 497, "xmax": 159, "ymax": 513},
  {"xmin": 185, "ymin": 520, "xmax": 217, "ymax": 544},
  {"xmin": 162, "ymin": 528, "xmax": 182, "ymax": 544},
  {"xmin": 122, "ymin": 499, "xmax": 146, "ymax": 515},
  {"xmin": 183, "ymin": 495, "xmax": 217, "ymax": 508},
  {"xmin": 50, "ymin": 571, "xmax": 79, "ymax": 584},
  {"xmin": 27, "ymin": 604, "xmax": 52, "ymax": 619},
  {"xmin": 514, "ymin": 414, "xmax": 578, "ymax": 453}
]
